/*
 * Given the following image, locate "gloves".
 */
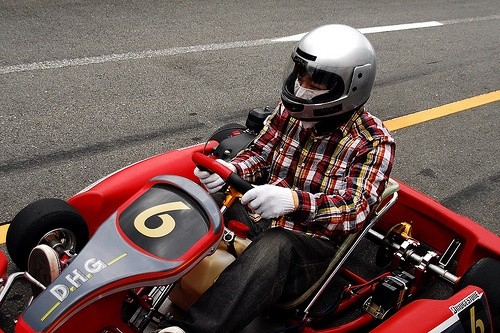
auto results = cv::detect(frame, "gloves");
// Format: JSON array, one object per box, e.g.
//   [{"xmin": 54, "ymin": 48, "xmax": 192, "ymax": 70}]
[
  {"xmin": 194, "ymin": 159, "xmax": 237, "ymax": 194},
  {"xmin": 240, "ymin": 184, "xmax": 297, "ymax": 219}
]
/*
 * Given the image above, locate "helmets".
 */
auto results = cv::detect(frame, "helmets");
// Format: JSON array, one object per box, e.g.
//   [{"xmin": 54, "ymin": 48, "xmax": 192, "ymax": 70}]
[{"xmin": 280, "ymin": 24, "xmax": 376, "ymax": 120}]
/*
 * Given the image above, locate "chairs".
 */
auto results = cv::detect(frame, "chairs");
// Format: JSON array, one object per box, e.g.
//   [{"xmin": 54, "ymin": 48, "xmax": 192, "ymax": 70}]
[{"xmin": 169, "ymin": 177, "xmax": 400, "ymax": 333}]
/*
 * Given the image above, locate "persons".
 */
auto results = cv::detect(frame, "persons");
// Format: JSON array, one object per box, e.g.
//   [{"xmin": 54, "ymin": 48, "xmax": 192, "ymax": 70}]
[{"xmin": 27, "ymin": 24, "xmax": 397, "ymax": 333}]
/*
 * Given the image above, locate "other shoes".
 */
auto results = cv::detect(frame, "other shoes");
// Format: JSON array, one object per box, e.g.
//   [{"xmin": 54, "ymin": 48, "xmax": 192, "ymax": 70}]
[
  {"xmin": 27, "ymin": 244, "xmax": 62, "ymax": 298},
  {"xmin": 160, "ymin": 326, "xmax": 184, "ymax": 333}
]
